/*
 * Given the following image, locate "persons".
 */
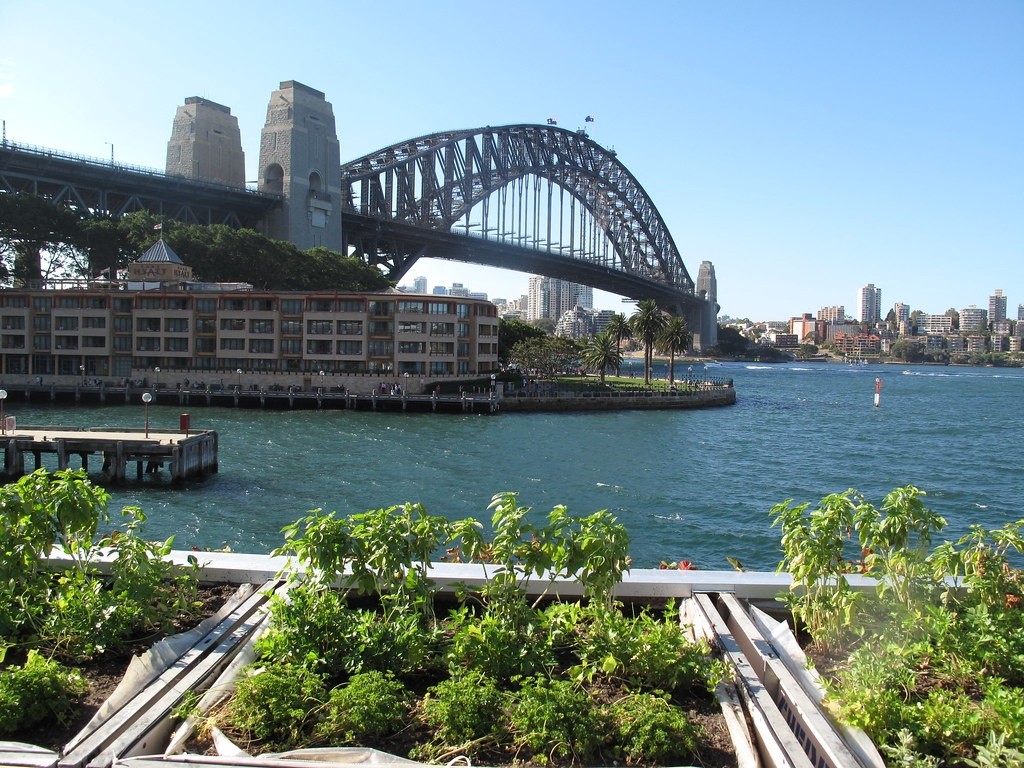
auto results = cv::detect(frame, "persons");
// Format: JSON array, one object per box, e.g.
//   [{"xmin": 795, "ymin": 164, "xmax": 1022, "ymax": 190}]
[
  {"xmin": 336, "ymin": 385, "xmax": 345, "ymax": 392},
  {"xmin": 288, "ymin": 384, "xmax": 301, "ymax": 392},
  {"xmin": 184, "ymin": 377, "xmax": 205, "ymax": 391},
  {"xmin": 272, "ymin": 383, "xmax": 281, "ymax": 392},
  {"xmin": 220, "ymin": 379, "xmax": 224, "ymax": 390},
  {"xmin": 436, "ymin": 385, "xmax": 440, "ymax": 397},
  {"xmin": 36, "ymin": 376, "xmax": 43, "ymax": 386},
  {"xmin": 378, "ymin": 382, "xmax": 401, "ymax": 395},
  {"xmin": 121, "ymin": 378, "xmax": 142, "ymax": 387},
  {"xmin": 248, "ymin": 383, "xmax": 258, "ymax": 391},
  {"xmin": 83, "ymin": 376, "xmax": 102, "ymax": 387}
]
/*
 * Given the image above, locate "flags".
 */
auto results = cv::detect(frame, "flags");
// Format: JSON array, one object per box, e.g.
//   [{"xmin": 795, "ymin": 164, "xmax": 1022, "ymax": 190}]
[
  {"xmin": 585, "ymin": 113, "xmax": 595, "ymax": 122},
  {"xmin": 547, "ymin": 116, "xmax": 557, "ymax": 125},
  {"xmin": 154, "ymin": 223, "xmax": 161, "ymax": 229}
]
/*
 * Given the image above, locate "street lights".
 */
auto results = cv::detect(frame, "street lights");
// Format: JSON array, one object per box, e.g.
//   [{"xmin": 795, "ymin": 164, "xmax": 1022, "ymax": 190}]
[
  {"xmin": 648, "ymin": 367, "xmax": 653, "ymax": 388},
  {"xmin": 687, "ymin": 367, "xmax": 694, "ymax": 386},
  {"xmin": 141, "ymin": 391, "xmax": 154, "ymax": 438},
  {"xmin": 628, "ymin": 362, "xmax": 633, "ymax": 377},
  {"xmin": 663, "ymin": 364, "xmax": 668, "ymax": 382},
  {"xmin": 0, "ymin": 389, "xmax": 9, "ymax": 437},
  {"xmin": 319, "ymin": 370, "xmax": 326, "ymax": 394},
  {"xmin": 154, "ymin": 366, "xmax": 161, "ymax": 388},
  {"xmin": 401, "ymin": 371, "xmax": 411, "ymax": 397},
  {"xmin": 236, "ymin": 367, "xmax": 243, "ymax": 393},
  {"xmin": 704, "ymin": 366, "xmax": 710, "ymax": 385},
  {"xmin": 486, "ymin": 372, "xmax": 499, "ymax": 400},
  {"xmin": 79, "ymin": 364, "xmax": 86, "ymax": 385}
]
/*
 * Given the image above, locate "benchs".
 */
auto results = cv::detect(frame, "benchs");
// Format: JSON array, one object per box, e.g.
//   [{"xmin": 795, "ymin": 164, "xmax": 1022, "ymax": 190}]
[
  {"xmin": 330, "ymin": 387, "xmax": 345, "ymax": 393},
  {"xmin": 312, "ymin": 386, "xmax": 327, "ymax": 393},
  {"xmin": 287, "ymin": 386, "xmax": 302, "ymax": 392},
  {"xmin": 210, "ymin": 384, "xmax": 220, "ymax": 389},
  {"xmin": 191, "ymin": 384, "xmax": 205, "ymax": 390},
  {"xmin": 268, "ymin": 385, "xmax": 283, "ymax": 392},
  {"xmin": 153, "ymin": 383, "xmax": 166, "ymax": 388},
  {"xmin": 228, "ymin": 384, "xmax": 242, "ymax": 390}
]
